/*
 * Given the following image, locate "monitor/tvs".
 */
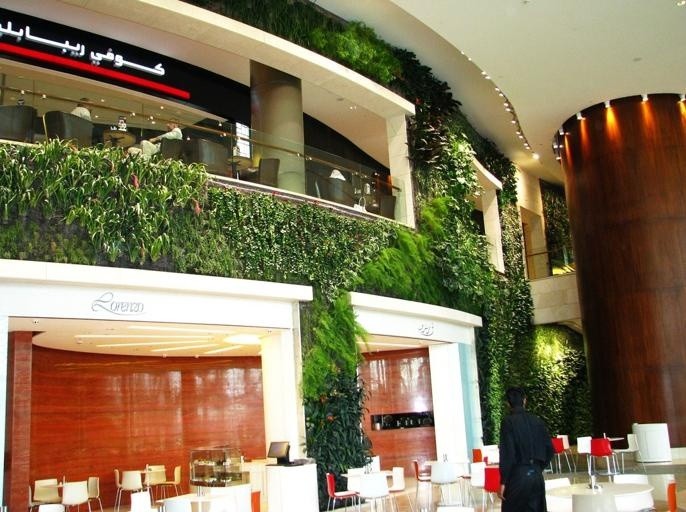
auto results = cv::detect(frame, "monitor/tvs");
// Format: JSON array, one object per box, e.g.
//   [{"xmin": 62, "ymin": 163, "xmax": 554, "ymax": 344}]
[{"xmin": 267, "ymin": 441, "xmax": 289, "ymax": 457}]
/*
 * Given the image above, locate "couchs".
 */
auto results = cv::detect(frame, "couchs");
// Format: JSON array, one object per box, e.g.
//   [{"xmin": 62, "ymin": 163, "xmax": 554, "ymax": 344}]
[
  {"xmin": 42, "ymin": 111, "xmax": 94, "ymax": 152},
  {"xmin": 192, "ymin": 139, "xmax": 232, "ymax": 177},
  {"xmin": 152, "ymin": 136, "xmax": 197, "ymax": 163},
  {"xmin": 2, "ymin": 103, "xmax": 38, "ymax": 143},
  {"xmin": 317, "ymin": 176, "xmax": 354, "ymax": 206},
  {"xmin": 240, "ymin": 157, "xmax": 281, "ymax": 186},
  {"xmin": 365, "ymin": 196, "xmax": 396, "ymax": 218}
]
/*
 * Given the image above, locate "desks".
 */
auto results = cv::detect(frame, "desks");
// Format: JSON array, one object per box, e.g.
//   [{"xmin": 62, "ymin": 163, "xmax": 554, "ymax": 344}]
[
  {"xmin": 102, "ymin": 129, "xmax": 135, "ymax": 152},
  {"xmin": 231, "ymin": 156, "xmax": 253, "ymax": 181}
]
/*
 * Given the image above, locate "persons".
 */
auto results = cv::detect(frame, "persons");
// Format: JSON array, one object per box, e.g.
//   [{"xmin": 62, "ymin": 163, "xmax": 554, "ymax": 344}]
[
  {"xmin": 128, "ymin": 118, "xmax": 183, "ymax": 157},
  {"xmin": 72, "ymin": 97, "xmax": 93, "ymax": 122},
  {"xmin": 498, "ymin": 386, "xmax": 554, "ymax": 512}
]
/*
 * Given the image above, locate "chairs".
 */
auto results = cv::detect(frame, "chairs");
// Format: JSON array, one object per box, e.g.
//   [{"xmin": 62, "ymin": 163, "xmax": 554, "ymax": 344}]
[{"xmin": 24, "ymin": 420, "xmax": 686, "ymax": 512}]
